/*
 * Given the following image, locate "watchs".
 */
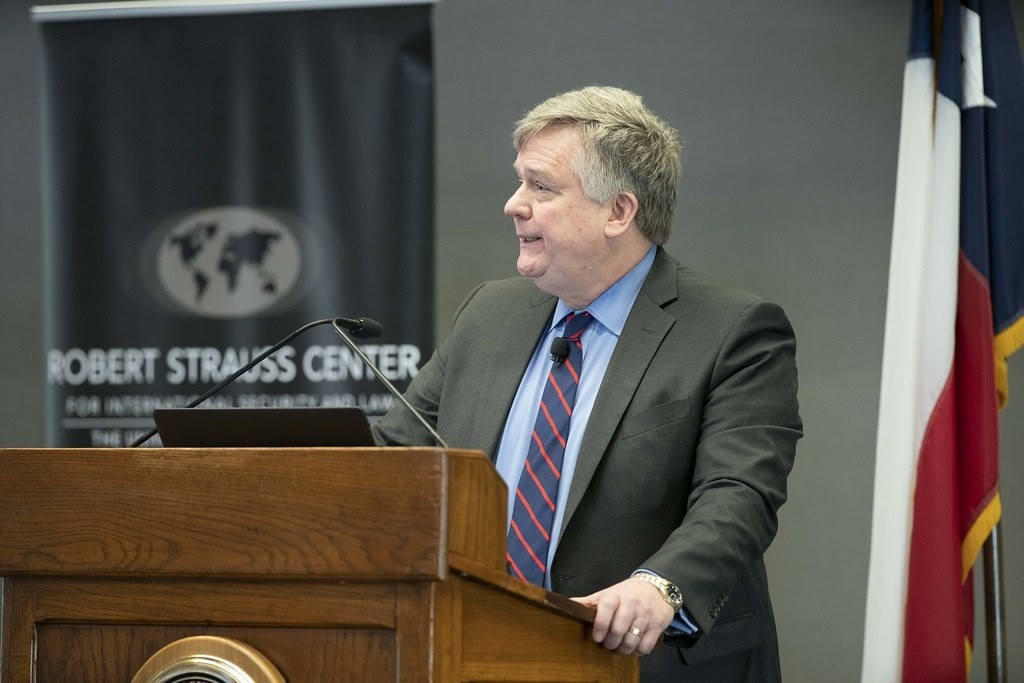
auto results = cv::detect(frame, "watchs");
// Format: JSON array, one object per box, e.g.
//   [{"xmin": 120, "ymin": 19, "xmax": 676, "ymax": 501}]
[{"xmin": 630, "ymin": 572, "xmax": 684, "ymax": 615}]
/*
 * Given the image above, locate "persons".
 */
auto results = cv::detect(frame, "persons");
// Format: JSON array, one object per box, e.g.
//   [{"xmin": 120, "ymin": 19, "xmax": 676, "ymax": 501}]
[{"xmin": 367, "ymin": 86, "xmax": 804, "ymax": 683}]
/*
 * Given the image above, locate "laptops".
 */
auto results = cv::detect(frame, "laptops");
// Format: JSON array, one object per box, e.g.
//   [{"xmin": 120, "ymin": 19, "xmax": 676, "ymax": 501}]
[{"xmin": 153, "ymin": 406, "xmax": 378, "ymax": 447}]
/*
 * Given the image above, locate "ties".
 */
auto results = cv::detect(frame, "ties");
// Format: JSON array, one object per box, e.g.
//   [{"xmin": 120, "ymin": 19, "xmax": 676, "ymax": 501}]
[{"xmin": 502, "ymin": 307, "xmax": 596, "ymax": 588}]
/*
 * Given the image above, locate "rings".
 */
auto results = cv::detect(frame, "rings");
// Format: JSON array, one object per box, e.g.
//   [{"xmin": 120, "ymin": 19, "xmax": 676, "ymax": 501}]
[{"xmin": 629, "ymin": 626, "xmax": 644, "ymax": 639}]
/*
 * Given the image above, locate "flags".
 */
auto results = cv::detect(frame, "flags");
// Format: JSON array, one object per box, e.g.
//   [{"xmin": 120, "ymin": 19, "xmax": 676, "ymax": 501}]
[{"xmin": 859, "ymin": 0, "xmax": 1023, "ymax": 682}]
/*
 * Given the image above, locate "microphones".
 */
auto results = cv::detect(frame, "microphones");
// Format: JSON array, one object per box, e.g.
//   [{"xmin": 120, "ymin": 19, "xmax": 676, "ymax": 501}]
[
  {"xmin": 332, "ymin": 317, "xmax": 451, "ymax": 448},
  {"xmin": 127, "ymin": 319, "xmax": 372, "ymax": 447}
]
